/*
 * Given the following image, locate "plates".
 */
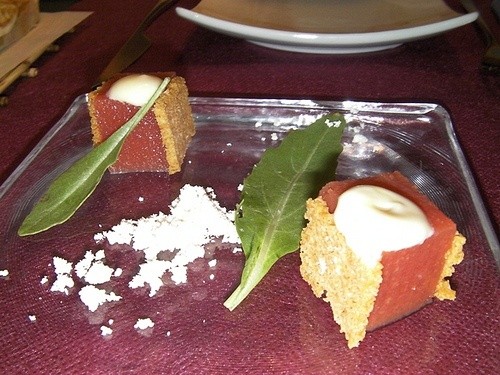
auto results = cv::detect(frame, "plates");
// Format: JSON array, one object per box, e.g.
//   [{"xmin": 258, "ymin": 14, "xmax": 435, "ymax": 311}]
[
  {"xmin": 1, "ymin": 92, "xmax": 499, "ymax": 375},
  {"xmin": 173, "ymin": 1, "xmax": 480, "ymax": 56}
]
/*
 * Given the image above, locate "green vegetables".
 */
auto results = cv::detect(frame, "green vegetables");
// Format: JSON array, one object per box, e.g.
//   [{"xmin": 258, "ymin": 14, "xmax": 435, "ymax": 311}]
[
  {"xmin": 222, "ymin": 110, "xmax": 346, "ymax": 310},
  {"xmin": 17, "ymin": 76, "xmax": 171, "ymax": 237}
]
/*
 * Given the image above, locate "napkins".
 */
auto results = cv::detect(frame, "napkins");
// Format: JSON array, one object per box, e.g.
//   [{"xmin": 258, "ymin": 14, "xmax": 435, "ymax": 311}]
[{"xmin": 0, "ymin": 12, "xmax": 96, "ymax": 79}]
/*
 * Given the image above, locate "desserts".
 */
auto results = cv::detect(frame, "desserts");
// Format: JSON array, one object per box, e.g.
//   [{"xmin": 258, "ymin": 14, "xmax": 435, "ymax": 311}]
[
  {"xmin": 299, "ymin": 170, "xmax": 466, "ymax": 351},
  {"xmin": 86, "ymin": 73, "xmax": 194, "ymax": 175}
]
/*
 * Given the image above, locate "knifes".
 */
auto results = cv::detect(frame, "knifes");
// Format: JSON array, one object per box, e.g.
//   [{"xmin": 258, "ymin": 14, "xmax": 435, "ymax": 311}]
[{"xmin": 89, "ymin": 0, "xmax": 173, "ymax": 89}]
